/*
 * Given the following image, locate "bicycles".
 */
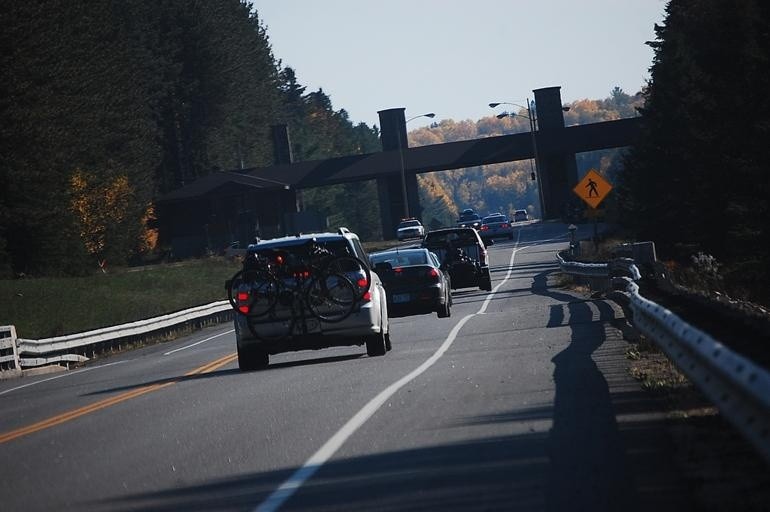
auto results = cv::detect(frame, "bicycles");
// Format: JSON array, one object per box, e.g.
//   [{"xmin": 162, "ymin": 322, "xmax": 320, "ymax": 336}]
[{"xmin": 224, "ymin": 244, "xmax": 370, "ymax": 323}]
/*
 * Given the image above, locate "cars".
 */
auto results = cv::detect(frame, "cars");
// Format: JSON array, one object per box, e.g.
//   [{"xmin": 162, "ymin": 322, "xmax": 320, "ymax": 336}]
[
  {"xmin": 369, "ymin": 246, "xmax": 452, "ymax": 319},
  {"xmin": 396, "ymin": 217, "xmax": 424, "ymax": 241},
  {"xmin": 456, "ymin": 208, "xmax": 529, "ymax": 241}
]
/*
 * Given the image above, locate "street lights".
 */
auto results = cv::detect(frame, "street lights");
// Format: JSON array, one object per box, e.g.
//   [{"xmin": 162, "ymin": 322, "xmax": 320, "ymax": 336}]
[
  {"xmin": 397, "ymin": 113, "xmax": 435, "ymax": 217},
  {"xmin": 489, "ymin": 97, "xmax": 570, "ymax": 222}
]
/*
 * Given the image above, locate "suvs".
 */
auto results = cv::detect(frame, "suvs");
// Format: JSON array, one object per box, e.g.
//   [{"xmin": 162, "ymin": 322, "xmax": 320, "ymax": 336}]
[
  {"xmin": 417, "ymin": 226, "xmax": 494, "ymax": 296},
  {"xmin": 225, "ymin": 225, "xmax": 392, "ymax": 368}
]
